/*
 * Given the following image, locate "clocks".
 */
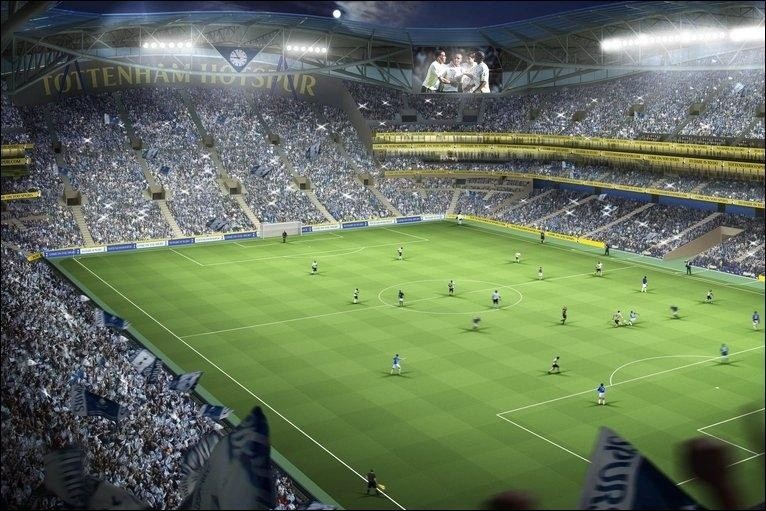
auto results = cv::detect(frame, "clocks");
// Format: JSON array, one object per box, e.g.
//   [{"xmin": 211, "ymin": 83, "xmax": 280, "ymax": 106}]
[{"xmin": 230, "ymin": 48, "xmax": 248, "ymax": 68}]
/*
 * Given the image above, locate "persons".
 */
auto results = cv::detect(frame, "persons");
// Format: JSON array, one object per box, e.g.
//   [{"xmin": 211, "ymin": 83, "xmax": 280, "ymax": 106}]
[
  {"xmin": 0, "ymin": 89, "xmax": 765, "ymax": 511},
  {"xmin": 578, "ymin": 45, "xmax": 765, "ymax": 87},
  {"xmin": 413, "ymin": 49, "xmax": 501, "ymax": 94}
]
